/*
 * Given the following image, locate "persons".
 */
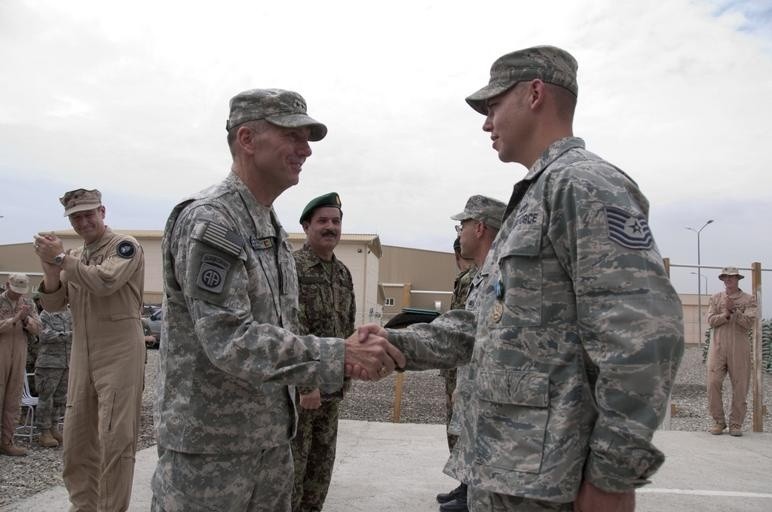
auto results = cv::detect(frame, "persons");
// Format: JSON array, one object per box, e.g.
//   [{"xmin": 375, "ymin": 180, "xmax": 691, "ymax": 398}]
[
  {"xmin": 346, "ymin": 44, "xmax": 683, "ymax": 512},
  {"xmin": 706, "ymin": 268, "xmax": 758, "ymax": 436},
  {"xmin": 289, "ymin": 191, "xmax": 356, "ymax": 512},
  {"xmin": 0, "ymin": 272, "xmax": 155, "ymax": 457},
  {"xmin": 450, "ymin": 195, "xmax": 508, "ymax": 309},
  {"xmin": 436, "ymin": 236, "xmax": 475, "ymax": 512},
  {"xmin": 36, "ymin": 188, "xmax": 144, "ymax": 512},
  {"xmin": 145, "ymin": 88, "xmax": 404, "ymax": 511}
]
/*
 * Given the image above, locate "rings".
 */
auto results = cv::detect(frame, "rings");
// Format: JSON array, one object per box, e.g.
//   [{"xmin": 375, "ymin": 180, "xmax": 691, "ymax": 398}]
[{"xmin": 379, "ymin": 366, "xmax": 388, "ymax": 378}]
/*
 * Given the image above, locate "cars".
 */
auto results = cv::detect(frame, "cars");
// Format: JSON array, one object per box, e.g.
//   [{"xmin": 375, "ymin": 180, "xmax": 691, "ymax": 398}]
[
  {"xmin": 141, "ymin": 308, "xmax": 162, "ymax": 346},
  {"xmin": 143, "ymin": 305, "xmax": 161, "ymax": 313}
]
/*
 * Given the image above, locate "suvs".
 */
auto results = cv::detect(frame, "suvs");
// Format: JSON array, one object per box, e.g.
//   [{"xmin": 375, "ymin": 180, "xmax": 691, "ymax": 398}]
[{"xmin": 381, "ymin": 307, "xmax": 443, "ymax": 329}]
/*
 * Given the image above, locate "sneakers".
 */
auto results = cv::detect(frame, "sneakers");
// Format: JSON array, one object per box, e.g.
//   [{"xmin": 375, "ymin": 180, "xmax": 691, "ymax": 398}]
[
  {"xmin": 39, "ymin": 431, "xmax": 62, "ymax": 447},
  {"xmin": 438, "ymin": 482, "xmax": 467, "ymax": 512},
  {"xmin": 729, "ymin": 424, "xmax": 743, "ymax": 436},
  {"xmin": 711, "ymin": 423, "xmax": 727, "ymax": 435},
  {"xmin": 0, "ymin": 444, "xmax": 26, "ymax": 456}
]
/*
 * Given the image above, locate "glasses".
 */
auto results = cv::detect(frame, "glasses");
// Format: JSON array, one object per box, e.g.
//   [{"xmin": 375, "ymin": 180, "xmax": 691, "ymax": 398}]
[{"xmin": 455, "ymin": 224, "xmax": 465, "ymax": 232}]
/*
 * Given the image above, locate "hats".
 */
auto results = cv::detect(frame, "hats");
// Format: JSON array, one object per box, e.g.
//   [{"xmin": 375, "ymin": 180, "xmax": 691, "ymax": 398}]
[
  {"xmin": 300, "ymin": 192, "xmax": 341, "ymax": 223},
  {"xmin": 226, "ymin": 88, "xmax": 326, "ymax": 141},
  {"xmin": 466, "ymin": 45, "xmax": 578, "ymax": 115},
  {"xmin": 7, "ymin": 273, "xmax": 29, "ymax": 294},
  {"xmin": 719, "ymin": 267, "xmax": 744, "ymax": 280},
  {"xmin": 451, "ymin": 195, "xmax": 507, "ymax": 229},
  {"xmin": 59, "ymin": 189, "xmax": 101, "ymax": 216}
]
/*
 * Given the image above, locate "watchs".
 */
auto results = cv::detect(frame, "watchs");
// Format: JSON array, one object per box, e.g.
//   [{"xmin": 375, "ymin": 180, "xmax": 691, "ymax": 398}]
[{"xmin": 54, "ymin": 252, "xmax": 66, "ymax": 267}]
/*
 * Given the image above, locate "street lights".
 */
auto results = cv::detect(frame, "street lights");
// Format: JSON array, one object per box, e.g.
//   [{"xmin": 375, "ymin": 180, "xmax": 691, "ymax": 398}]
[
  {"xmin": 684, "ymin": 216, "xmax": 714, "ymax": 349},
  {"xmin": 688, "ymin": 271, "xmax": 708, "ymax": 294}
]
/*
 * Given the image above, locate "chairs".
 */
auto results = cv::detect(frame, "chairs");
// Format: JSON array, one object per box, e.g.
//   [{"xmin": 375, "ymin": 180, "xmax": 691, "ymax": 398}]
[{"xmin": 14, "ymin": 367, "xmax": 64, "ymax": 443}]
